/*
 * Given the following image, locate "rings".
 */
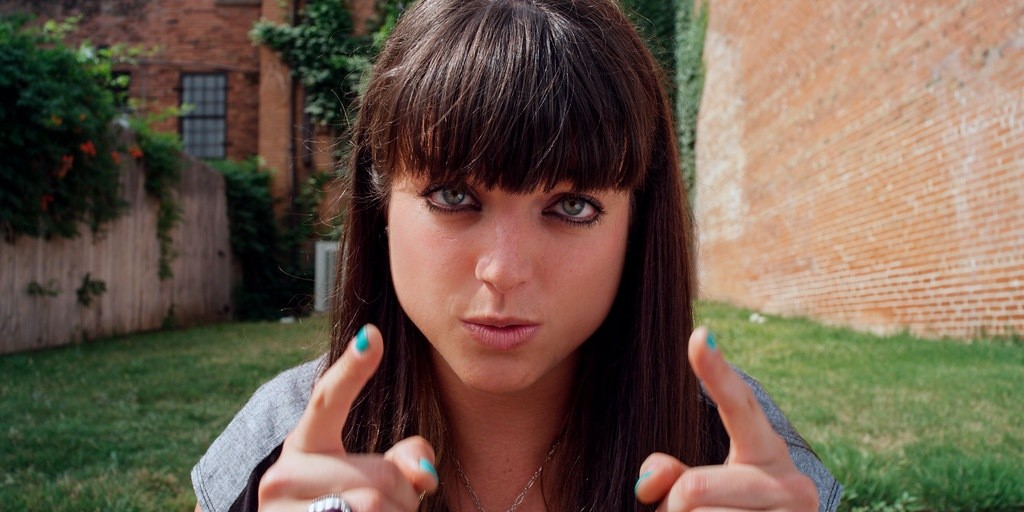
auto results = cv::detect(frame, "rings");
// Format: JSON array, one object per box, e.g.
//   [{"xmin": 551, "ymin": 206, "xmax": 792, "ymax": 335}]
[{"xmin": 308, "ymin": 495, "xmax": 350, "ymax": 512}]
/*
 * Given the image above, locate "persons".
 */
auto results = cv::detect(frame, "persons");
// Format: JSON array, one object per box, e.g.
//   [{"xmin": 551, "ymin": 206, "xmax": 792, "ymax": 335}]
[{"xmin": 191, "ymin": 0, "xmax": 844, "ymax": 512}]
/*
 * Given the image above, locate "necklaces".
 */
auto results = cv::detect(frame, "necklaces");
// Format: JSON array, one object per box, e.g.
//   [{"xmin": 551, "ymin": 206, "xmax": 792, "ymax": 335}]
[{"xmin": 440, "ymin": 412, "xmax": 566, "ymax": 512}]
